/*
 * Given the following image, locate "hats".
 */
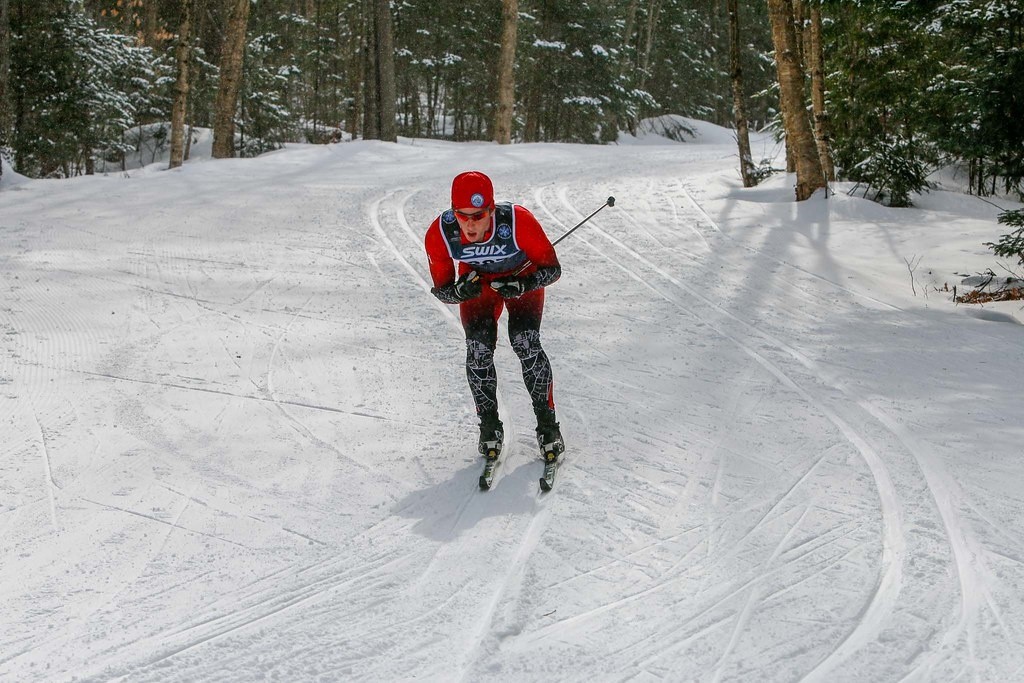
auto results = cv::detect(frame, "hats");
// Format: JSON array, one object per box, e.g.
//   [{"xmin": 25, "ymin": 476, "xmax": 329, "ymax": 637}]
[{"xmin": 452, "ymin": 171, "xmax": 493, "ymax": 208}]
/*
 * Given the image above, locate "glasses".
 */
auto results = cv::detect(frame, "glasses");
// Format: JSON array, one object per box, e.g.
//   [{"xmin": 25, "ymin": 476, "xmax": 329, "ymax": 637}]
[{"xmin": 454, "ymin": 204, "xmax": 490, "ymax": 221}]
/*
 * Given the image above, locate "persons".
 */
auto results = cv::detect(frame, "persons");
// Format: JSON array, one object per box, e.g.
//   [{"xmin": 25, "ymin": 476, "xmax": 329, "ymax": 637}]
[{"xmin": 424, "ymin": 173, "xmax": 566, "ymax": 464}]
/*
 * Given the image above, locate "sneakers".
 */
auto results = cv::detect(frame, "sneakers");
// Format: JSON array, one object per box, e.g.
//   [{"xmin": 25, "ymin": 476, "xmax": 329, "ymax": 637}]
[
  {"xmin": 478, "ymin": 420, "xmax": 504, "ymax": 458},
  {"xmin": 535, "ymin": 423, "xmax": 565, "ymax": 458}
]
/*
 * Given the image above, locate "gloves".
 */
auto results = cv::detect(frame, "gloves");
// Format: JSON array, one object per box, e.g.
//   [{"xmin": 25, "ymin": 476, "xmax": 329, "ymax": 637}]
[
  {"xmin": 487, "ymin": 276, "xmax": 530, "ymax": 299},
  {"xmin": 454, "ymin": 270, "xmax": 482, "ymax": 300}
]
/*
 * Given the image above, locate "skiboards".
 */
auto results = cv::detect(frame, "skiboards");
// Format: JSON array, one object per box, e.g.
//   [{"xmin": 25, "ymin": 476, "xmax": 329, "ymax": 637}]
[{"xmin": 478, "ymin": 441, "xmax": 560, "ymax": 492}]
